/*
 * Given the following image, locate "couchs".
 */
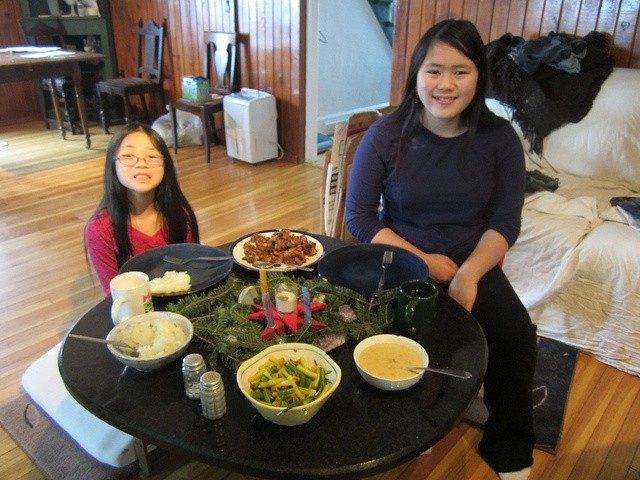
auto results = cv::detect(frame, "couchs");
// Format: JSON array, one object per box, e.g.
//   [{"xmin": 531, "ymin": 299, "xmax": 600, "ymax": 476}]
[{"xmin": 485, "ymin": 66, "xmax": 639, "ymax": 377}]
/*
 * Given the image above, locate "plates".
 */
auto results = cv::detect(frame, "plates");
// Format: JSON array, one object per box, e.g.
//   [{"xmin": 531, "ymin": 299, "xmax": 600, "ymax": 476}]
[
  {"xmin": 317, "ymin": 242, "xmax": 429, "ymax": 301},
  {"xmin": 229, "ymin": 228, "xmax": 327, "ymax": 273},
  {"xmin": 117, "ymin": 242, "xmax": 233, "ymax": 303}
]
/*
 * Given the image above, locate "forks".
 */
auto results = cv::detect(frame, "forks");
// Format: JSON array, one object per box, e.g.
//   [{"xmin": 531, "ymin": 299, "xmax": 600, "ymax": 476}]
[
  {"xmin": 377, "ymin": 249, "xmax": 394, "ymax": 291},
  {"xmin": 161, "ymin": 255, "xmax": 234, "ymax": 266}
]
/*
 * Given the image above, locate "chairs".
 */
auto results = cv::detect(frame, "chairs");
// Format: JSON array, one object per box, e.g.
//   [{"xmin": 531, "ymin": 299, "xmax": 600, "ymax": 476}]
[
  {"xmin": 170, "ymin": 31, "xmax": 245, "ymax": 161},
  {"xmin": 19, "ymin": 18, "xmax": 92, "ymax": 140},
  {"xmin": 95, "ymin": 19, "xmax": 168, "ymax": 135}
]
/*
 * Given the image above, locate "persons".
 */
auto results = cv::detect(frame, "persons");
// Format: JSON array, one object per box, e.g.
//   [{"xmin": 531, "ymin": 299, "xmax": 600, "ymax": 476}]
[
  {"xmin": 83, "ymin": 123, "xmax": 201, "ymax": 300},
  {"xmin": 341, "ymin": 17, "xmax": 538, "ymax": 480}
]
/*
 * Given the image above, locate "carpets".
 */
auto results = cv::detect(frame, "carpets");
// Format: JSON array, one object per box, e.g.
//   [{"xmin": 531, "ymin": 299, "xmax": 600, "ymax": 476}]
[{"xmin": 462, "ymin": 336, "xmax": 579, "ymax": 456}]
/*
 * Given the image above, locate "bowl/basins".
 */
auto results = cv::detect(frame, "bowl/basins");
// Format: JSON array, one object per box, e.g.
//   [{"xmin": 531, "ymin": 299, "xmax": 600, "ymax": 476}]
[
  {"xmin": 105, "ymin": 311, "xmax": 194, "ymax": 373},
  {"xmin": 353, "ymin": 334, "xmax": 429, "ymax": 392},
  {"xmin": 235, "ymin": 342, "xmax": 343, "ymax": 427}
]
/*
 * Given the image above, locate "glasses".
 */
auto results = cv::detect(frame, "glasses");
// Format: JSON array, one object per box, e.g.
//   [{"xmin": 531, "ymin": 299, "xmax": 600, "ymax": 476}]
[{"xmin": 114, "ymin": 152, "xmax": 164, "ymax": 167}]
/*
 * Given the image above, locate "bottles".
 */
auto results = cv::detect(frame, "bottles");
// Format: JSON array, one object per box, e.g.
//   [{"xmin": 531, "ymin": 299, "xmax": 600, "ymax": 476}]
[
  {"xmin": 197, "ymin": 371, "xmax": 228, "ymax": 421},
  {"xmin": 181, "ymin": 353, "xmax": 208, "ymax": 401}
]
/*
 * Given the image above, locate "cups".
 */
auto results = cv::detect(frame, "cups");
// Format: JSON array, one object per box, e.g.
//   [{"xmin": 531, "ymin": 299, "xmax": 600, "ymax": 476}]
[
  {"xmin": 109, "ymin": 271, "xmax": 156, "ymax": 327},
  {"xmin": 396, "ymin": 279, "xmax": 440, "ymax": 339},
  {"xmin": 274, "ymin": 281, "xmax": 298, "ymax": 314}
]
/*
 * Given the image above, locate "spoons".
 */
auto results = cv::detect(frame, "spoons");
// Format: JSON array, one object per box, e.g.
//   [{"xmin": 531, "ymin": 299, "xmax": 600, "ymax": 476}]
[{"xmin": 249, "ymin": 260, "xmax": 315, "ymax": 275}]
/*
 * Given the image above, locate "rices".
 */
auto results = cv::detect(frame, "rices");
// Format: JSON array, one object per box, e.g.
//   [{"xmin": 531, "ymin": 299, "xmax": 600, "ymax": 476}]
[
  {"xmin": 106, "ymin": 311, "xmax": 193, "ymax": 361},
  {"xmin": 150, "ymin": 270, "xmax": 192, "ymax": 294}
]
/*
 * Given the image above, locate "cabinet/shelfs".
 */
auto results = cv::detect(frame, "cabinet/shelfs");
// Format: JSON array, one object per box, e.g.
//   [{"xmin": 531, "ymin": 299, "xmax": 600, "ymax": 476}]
[{"xmin": 18, "ymin": 0, "xmax": 124, "ymax": 124}]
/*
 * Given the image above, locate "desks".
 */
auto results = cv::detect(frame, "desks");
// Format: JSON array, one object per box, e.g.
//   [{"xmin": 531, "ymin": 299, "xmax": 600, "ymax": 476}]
[{"xmin": 0, "ymin": 45, "xmax": 110, "ymax": 150}]
